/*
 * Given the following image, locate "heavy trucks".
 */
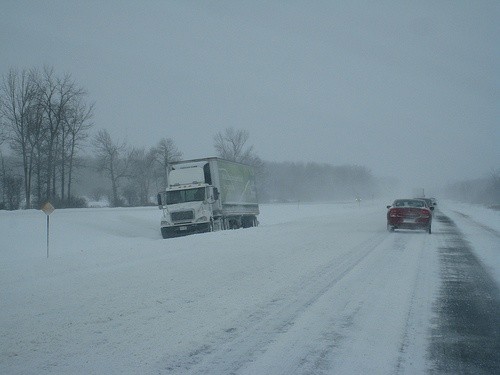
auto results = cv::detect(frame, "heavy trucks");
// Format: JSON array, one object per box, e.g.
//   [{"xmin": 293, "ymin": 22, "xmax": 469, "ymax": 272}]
[{"xmin": 156, "ymin": 156, "xmax": 263, "ymax": 239}]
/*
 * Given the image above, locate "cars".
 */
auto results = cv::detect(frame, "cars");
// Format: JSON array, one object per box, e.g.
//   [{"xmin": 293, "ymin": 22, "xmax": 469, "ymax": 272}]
[
  {"xmin": 414, "ymin": 197, "xmax": 437, "ymax": 210},
  {"xmin": 385, "ymin": 199, "xmax": 433, "ymax": 235}
]
